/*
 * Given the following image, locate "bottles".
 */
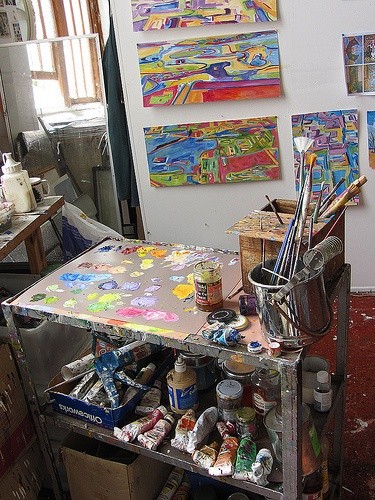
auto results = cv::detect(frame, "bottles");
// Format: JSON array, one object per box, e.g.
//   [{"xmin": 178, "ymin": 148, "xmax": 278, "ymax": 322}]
[
  {"xmin": 250, "ymin": 367, "xmax": 281, "ymax": 416},
  {"xmin": 313, "ymin": 371, "xmax": 332, "ymax": 412},
  {"xmin": 167, "ymin": 355, "xmax": 199, "ymax": 415},
  {"xmin": 1, "ymin": 153, "xmax": 36, "ymax": 212}
]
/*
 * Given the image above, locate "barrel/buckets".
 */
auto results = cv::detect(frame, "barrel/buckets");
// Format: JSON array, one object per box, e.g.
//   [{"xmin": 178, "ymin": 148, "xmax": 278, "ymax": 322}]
[{"xmin": 248, "ymin": 256, "xmax": 334, "ymax": 349}]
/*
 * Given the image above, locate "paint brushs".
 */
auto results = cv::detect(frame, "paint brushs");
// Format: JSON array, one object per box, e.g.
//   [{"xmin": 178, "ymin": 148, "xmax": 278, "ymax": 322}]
[
  {"xmin": 265, "ymin": 195, "xmax": 284, "ymax": 225},
  {"xmin": 228, "ymin": 286, "xmax": 243, "ymax": 300},
  {"xmin": 12, "ymin": 212, "xmax": 48, "ymax": 215},
  {"xmin": 312, "ymin": 175, "xmax": 368, "ymax": 224},
  {"xmin": 270, "ymin": 136, "xmax": 318, "ymax": 286}
]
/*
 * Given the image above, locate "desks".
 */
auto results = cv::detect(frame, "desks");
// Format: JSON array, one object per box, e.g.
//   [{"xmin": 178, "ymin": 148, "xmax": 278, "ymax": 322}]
[{"xmin": 0, "ymin": 195, "xmax": 64, "ymax": 275}]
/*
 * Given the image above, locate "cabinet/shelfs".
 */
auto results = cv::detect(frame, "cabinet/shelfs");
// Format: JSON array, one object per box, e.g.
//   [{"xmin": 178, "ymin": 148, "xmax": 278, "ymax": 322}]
[{"xmin": 1, "ymin": 235, "xmax": 352, "ymax": 500}]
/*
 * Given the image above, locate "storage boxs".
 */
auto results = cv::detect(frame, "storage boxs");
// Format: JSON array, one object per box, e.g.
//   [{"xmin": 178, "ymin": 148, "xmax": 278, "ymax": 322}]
[
  {"xmin": 91, "ymin": 330, "xmax": 160, "ymax": 377},
  {"xmin": 0, "ymin": 343, "xmax": 48, "ymax": 500},
  {"xmin": 224, "ymin": 196, "xmax": 348, "ymax": 297},
  {"xmin": 45, "ymin": 348, "xmax": 174, "ymax": 431},
  {"xmin": 0, "ymin": 268, "xmax": 76, "ymax": 385},
  {"xmin": 59, "ymin": 432, "xmax": 173, "ymax": 500}
]
